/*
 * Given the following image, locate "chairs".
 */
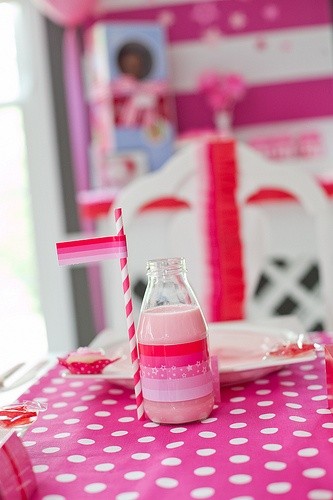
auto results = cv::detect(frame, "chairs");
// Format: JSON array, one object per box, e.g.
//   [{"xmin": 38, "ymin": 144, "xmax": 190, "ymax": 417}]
[{"xmin": 100, "ymin": 135, "xmax": 333, "ymax": 332}]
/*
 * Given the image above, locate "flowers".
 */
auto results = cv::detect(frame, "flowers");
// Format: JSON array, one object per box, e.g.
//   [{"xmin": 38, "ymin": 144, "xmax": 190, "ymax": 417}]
[{"xmin": 200, "ymin": 74, "xmax": 247, "ymax": 111}]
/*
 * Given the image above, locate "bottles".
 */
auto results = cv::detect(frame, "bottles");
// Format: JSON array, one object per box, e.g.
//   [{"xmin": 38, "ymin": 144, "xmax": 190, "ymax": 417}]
[{"xmin": 138, "ymin": 257, "xmax": 215, "ymax": 424}]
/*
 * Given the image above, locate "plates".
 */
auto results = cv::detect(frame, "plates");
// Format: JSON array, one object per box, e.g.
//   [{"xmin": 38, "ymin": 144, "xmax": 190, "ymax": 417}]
[{"xmin": 60, "ymin": 330, "xmax": 316, "ymax": 387}]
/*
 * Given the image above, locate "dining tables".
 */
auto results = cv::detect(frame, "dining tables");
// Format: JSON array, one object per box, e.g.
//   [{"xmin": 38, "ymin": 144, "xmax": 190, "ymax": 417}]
[{"xmin": 0, "ymin": 333, "xmax": 333, "ymax": 499}]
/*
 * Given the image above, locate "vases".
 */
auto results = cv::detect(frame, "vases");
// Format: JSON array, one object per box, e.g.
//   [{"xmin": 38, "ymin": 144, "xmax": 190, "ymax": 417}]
[{"xmin": 215, "ymin": 109, "xmax": 233, "ymax": 135}]
